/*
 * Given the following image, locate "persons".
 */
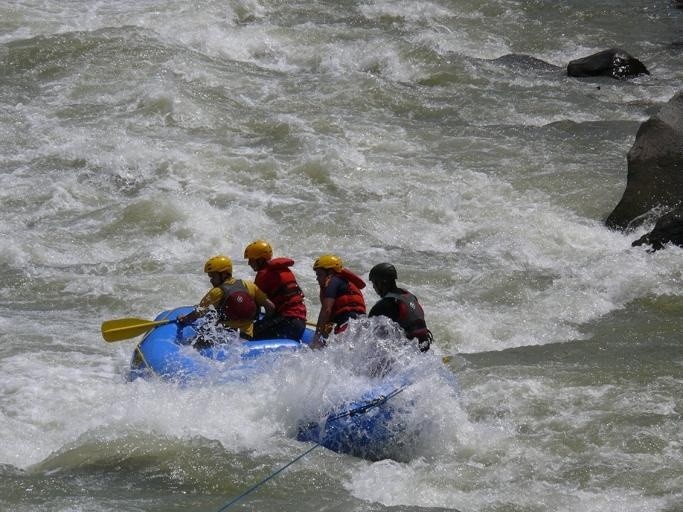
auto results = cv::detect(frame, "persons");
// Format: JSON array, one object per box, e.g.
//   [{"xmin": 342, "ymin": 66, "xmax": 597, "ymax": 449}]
[
  {"xmin": 368, "ymin": 262, "xmax": 433, "ymax": 352},
  {"xmin": 241, "ymin": 239, "xmax": 307, "ymax": 341},
  {"xmin": 309, "ymin": 255, "xmax": 366, "ymax": 344},
  {"xmin": 175, "ymin": 255, "xmax": 276, "ymax": 348}
]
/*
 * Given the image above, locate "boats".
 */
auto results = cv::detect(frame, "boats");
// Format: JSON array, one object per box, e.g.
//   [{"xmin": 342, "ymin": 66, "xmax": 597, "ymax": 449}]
[{"xmin": 126, "ymin": 304, "xmax": 460, "ymax": 452}]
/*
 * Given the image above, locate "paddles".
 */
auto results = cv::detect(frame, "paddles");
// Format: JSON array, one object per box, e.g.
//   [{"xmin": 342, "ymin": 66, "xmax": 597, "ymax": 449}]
[{"xmin": 101, "ymin": 316, "xmax": 178, "ymax": 342}]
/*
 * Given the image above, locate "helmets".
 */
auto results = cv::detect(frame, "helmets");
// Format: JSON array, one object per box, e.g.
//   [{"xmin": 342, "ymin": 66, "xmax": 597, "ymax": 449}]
[
  {"xmin": 244, "ymin": 240, "xmax": 273, "ymax": 261},
  {"xmin": 369, "ymin": 263, "xmax": 397, "ymax": 283},
  {"xmin": 313, "ymin": 255, "xmax": 342, "ymax": 273},
  {"xmin": 205, "ymin": 256, "xmax": 232, "ymax": 277}
]
